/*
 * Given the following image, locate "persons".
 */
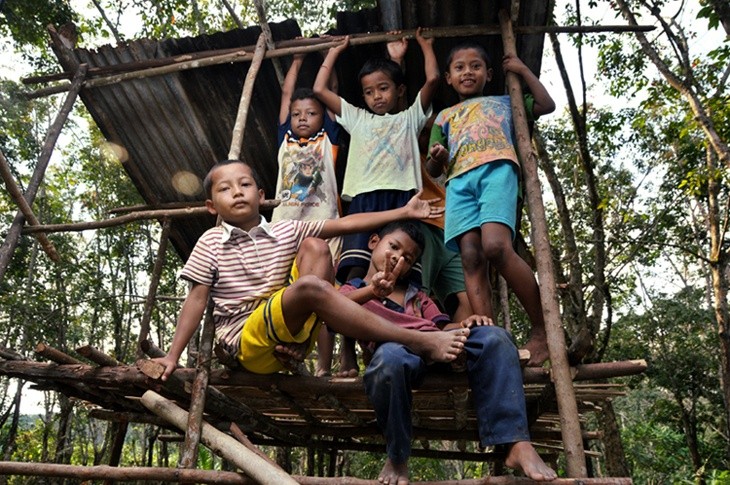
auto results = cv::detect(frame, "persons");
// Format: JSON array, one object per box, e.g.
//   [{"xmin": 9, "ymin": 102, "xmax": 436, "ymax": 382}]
[
  {"xmin": 313, "ymin": 25, "xmax": 440, "ymax": 377},
  {"xmin": 425, "ymin": 43, "xmax": 556, "ymax": 367},
  {"xmin": 150, "ymin": 160, "xmax": 471, "ymax": 392},
  {"xmin": 385, "ymin": 30, "xmax": 472, "ymax": 323},
  {"xmin": 338, "ymin": 220, "xmax": 557, "ymax": 485},
  {"xmin": 271, "ymin": 34, "xmax": 344, "ymax": 377}
]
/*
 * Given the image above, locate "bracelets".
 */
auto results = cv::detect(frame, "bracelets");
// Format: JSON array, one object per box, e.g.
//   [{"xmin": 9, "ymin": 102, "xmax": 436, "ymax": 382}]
[{"xmin": 320, "ymin": 65, "xmax": 329, "ymax": 70}]
